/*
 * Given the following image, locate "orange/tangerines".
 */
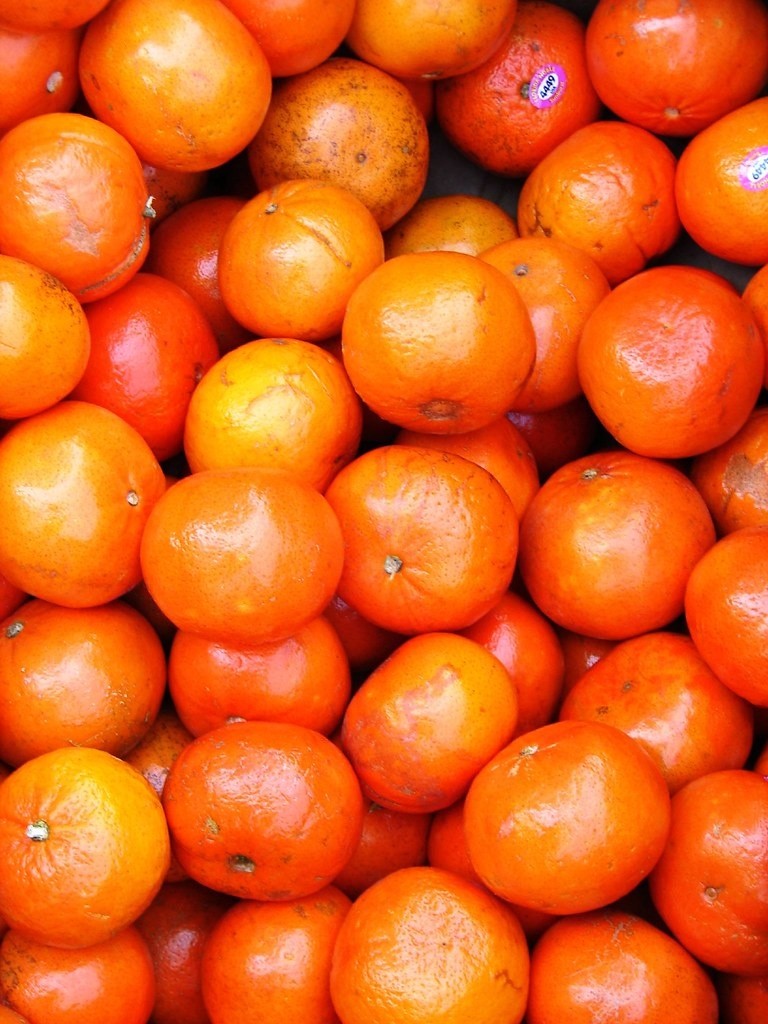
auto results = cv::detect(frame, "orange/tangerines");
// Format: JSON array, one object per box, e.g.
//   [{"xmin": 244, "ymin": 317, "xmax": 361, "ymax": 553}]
[{"xmin": 0, "ymin": 0, "xmax": 768, "ymax": 1024}]
